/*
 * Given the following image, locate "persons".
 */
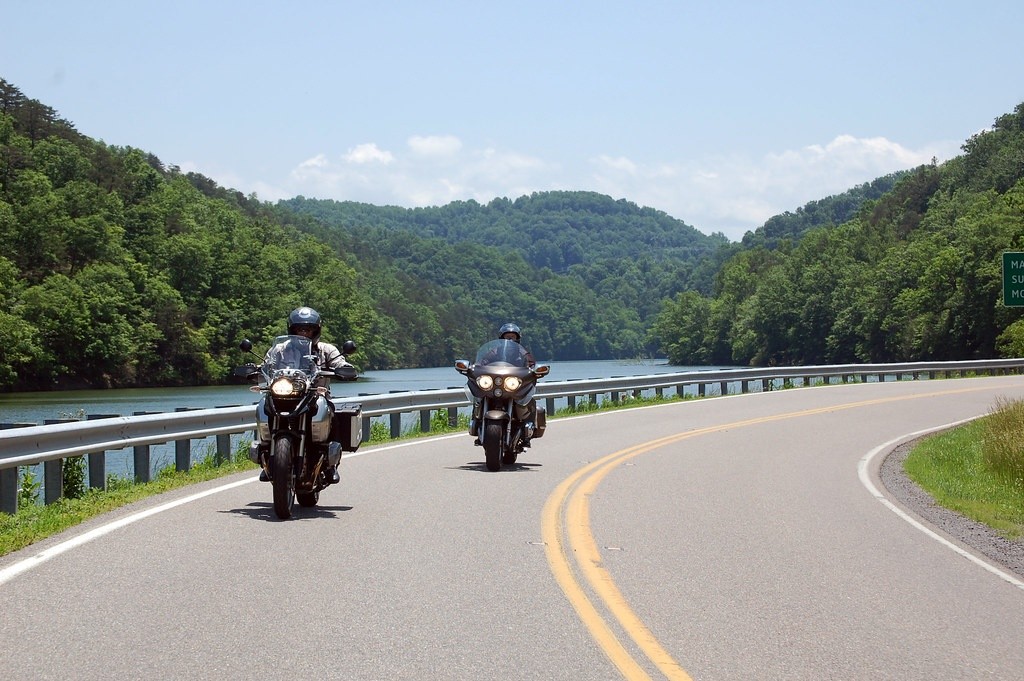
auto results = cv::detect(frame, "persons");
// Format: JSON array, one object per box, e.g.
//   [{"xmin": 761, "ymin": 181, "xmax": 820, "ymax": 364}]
[
  {"xmin": 474, "ymin": 322, "xmax": 535, "ymax": 448},
  {"xmin": 245, "ymin": 307, "xmax": 358, "ymax": 484}
]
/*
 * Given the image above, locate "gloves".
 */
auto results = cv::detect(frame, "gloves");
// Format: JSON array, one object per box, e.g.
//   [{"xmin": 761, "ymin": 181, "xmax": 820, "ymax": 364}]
[
  {"xmin": 340, "ymin": 364, "xmax": 355, "ymax": 381},
  {"xmin": 245, "ymin": 363, "xmax": 257, "ymax": 379}
]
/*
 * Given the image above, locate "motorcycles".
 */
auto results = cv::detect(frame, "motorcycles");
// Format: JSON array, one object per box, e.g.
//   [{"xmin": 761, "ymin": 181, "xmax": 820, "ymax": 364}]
[
  {"xmin": 240, "ymin": 335, "xmax": 364, "ymax": 519},
  {"xmin": 455, "ymin": 339, "xmax": 550, "ymax": 472}
]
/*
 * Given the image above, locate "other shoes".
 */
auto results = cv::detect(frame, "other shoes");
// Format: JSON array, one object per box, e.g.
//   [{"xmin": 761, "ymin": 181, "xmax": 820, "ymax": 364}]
[
  {"xmin": 522, "ymin": 440, "xmax": 531, "ymax": 448},
  {"xmin": 473, "ymin": 438, "xmax": 482, "ymax": 446},
  {"xmin": 259, "ymin": 470, "xmax": 269, "ymax": 482},
  {"xmin": 325, "ymin": 470, "xmax": 340, "ymax": 484}
]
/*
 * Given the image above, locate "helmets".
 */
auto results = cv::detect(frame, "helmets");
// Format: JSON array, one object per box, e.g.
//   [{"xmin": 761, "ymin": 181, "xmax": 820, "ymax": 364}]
[
  {"xmin": 498, "ymin": 323, "xmax": 521, "ymax": 345},
  {"xmin": 287, "ymin": 307, "xmax": 322, "ymax": 346}
]
